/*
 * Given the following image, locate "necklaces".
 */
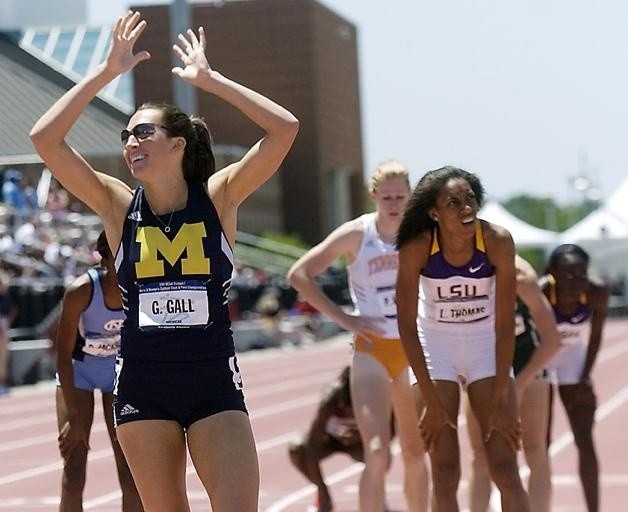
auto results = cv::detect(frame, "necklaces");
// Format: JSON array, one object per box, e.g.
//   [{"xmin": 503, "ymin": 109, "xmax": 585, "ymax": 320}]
[{"xmin": 139, "ymin": 188, "xmax": 186, "ymax": 232}]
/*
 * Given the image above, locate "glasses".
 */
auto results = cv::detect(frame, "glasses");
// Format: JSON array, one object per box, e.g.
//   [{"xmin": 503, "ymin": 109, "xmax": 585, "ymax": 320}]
[{"xmin": 121, "ymin": 122, "xmax": 171, "ymax": 142}]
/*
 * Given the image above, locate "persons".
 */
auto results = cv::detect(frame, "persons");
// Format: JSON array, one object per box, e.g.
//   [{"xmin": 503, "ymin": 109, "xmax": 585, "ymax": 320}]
[
  {"xmin": 29, "ymin": 9, "xmax": 300, "ymax": 512},
  {"xmin": 1, "ymin": 166, "xmax": 348, "ymax": 384},
  {"xmin": 54, "ymin": 228, "xmax": 142, "ymax": 512},
  {"xmin": 288, "ymin": 161, "xmax": 610, "ymax": 511}
]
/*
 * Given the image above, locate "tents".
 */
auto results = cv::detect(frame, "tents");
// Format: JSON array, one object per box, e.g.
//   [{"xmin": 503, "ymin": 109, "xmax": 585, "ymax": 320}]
[{"xmin": 471, "ymin": 179, "xmax": 627, "ymax": 295}]
